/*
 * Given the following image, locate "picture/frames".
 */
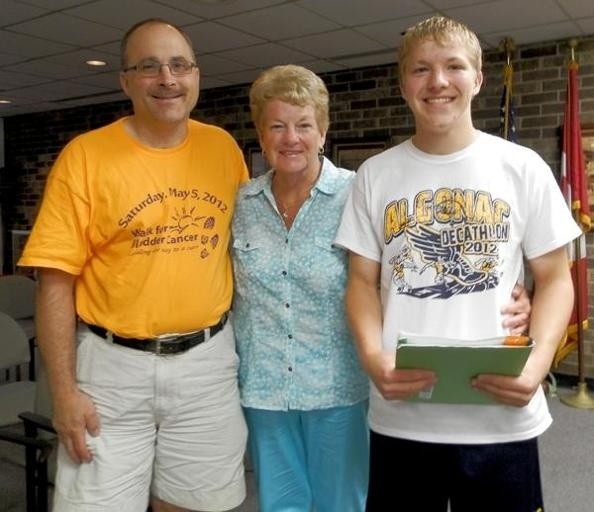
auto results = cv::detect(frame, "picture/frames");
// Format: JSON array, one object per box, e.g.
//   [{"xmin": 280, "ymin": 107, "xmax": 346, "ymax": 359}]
[
  {"xmin": 251, "ymin": 148, "xmax": 261, "ymax": 176},
  {"xmin": 557, "ymin": 124, "xmax": 594, "ymax": 212},
  {"xmin": 334, "ymin": 141, "xmax": 384, "ymax": 170}
]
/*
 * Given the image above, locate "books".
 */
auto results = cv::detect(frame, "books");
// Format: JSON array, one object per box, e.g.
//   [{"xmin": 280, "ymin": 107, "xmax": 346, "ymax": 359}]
[{"xmin": 394, "ymin": 331, "xmax": 536, "ymax": 404}]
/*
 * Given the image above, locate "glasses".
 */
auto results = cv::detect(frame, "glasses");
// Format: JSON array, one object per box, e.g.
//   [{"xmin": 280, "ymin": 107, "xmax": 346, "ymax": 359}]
[{"xmin": 124, "ymin": 59, "xmax": 201, "ymax": 78}]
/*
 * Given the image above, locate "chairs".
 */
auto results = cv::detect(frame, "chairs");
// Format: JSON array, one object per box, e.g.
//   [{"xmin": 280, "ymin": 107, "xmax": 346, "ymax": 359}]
[{"xmin": 1, "ymin": 274, "xmax": 59, "ymax": 511}]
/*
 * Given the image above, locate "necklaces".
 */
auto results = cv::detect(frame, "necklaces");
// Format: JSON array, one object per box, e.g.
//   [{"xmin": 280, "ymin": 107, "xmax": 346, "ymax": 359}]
[{"xmin": 271, "ymin": 185, "xmax": 311, "ymax": 218}]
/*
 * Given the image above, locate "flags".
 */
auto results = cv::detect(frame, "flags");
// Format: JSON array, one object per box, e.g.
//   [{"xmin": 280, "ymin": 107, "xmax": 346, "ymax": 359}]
[
  {"xmin": 500, "ymin": 63, "xmax": 518, "ymax": 143},
  {"xmin": 555, "ymin": 60, "xmax": 591, "ymax": 367}
]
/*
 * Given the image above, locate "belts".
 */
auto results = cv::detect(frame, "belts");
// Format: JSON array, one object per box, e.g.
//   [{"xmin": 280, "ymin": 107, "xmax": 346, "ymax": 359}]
[{"xmin": 88, "ymin": 311, "xmax": 228, "ymax": 356}]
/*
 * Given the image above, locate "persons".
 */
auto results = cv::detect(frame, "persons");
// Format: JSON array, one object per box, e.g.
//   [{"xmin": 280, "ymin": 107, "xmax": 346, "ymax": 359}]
[
  {"xmin": 230, "ymin": 63, "xmax": 531, "ymax": 512},
  {"xmin": 332, "ymin": 14, "xmax": 583, "ymax": 512},
  {"xmin": 16, "ymin": 19, "xmax": 251, "ymax": 512}
]
